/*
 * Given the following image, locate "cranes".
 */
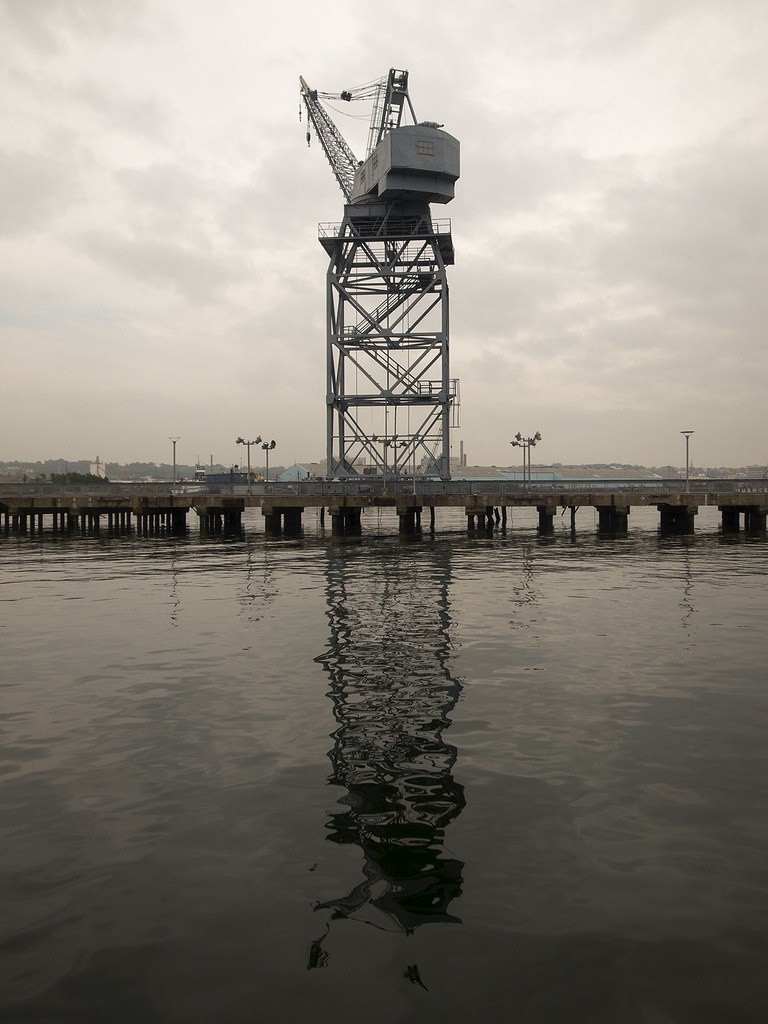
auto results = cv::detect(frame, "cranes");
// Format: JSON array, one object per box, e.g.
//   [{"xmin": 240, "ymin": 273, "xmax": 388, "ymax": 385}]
[{"xmin": 298, "ymin": 68, "xmax": 460, "ymax": 482}]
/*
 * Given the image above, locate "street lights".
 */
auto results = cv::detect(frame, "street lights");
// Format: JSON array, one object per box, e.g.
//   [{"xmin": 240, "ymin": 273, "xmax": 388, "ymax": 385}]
[
  {"xmin": 510, "ymin": 441, "xmax": 536, "ymax": 487},
  {"xmin": 235, "ymin": 435, "xmax": 262, "ymax": 496},
  {"xmin": 370, "ymin": 434, "xmax": 408, "ymax": 495},
  {"xmin": 170, "ymin": 436, "xmax": 180, "ymax": 495},
  {"xmin": 681, "ymin": 431, "xmax": 694, "ymax": 493},
  {"xmin": 261, "ymin": 440, "xmax": 276, "ymax": 482},
  {"xmin": 515, "ymin": 431, "xmax": 541, "ymax": 487}
]
